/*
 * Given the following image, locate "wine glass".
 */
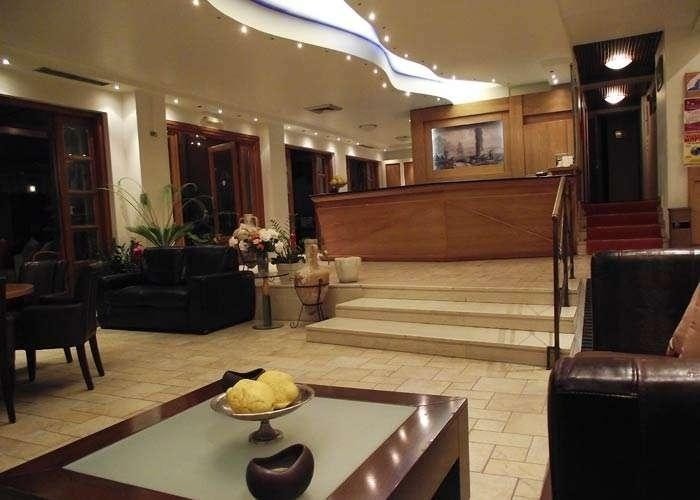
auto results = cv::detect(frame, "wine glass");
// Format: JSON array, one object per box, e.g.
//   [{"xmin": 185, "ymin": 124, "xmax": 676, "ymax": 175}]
[{"xmin": 209, "ymin": 382, "xmax": 315, "ymax": 447}]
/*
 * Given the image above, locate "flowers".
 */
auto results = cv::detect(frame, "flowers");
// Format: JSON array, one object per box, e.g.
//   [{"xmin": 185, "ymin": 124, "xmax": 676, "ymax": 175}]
[{"xmin": 231, "ymin": 222, "xmax": 280, "ymax": 268}]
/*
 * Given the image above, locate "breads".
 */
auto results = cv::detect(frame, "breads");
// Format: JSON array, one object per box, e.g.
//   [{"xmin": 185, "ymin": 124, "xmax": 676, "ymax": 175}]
[{"xmin": 228, "ymin": 370, "xmax": 299, "ymax": 411}]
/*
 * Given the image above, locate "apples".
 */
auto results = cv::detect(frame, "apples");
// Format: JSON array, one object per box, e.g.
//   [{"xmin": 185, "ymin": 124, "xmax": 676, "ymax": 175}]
[{"xmin": 330, "ymin": 175, "xmax": 344, "ymax": 186}]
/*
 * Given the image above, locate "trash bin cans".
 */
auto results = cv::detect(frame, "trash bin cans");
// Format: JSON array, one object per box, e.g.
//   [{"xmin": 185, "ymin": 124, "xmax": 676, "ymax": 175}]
[{"xmin": 667, "ymin": 207, "xmax": 691, "ymax": 248}]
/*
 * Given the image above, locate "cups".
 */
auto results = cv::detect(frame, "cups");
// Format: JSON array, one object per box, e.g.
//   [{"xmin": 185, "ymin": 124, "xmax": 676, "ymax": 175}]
[{"xmin": 333, "ymin": 257, "xmax": 362, "ymax": 283}]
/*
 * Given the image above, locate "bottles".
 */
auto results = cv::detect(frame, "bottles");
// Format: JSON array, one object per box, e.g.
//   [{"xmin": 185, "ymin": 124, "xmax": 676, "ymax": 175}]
[{"xmin": 293, "ymin": 240, "xmax": 330, "ymax": 306}]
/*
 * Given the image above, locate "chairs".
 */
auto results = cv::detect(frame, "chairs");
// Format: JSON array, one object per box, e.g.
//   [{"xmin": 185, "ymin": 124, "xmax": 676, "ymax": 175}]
[
  {"xmin": 1, "ymin": 245, "xmax": 105, "ymax": 424},
  {"xmin": 548, "ymin": 250, "xmax": 700, "ymax": 498}
]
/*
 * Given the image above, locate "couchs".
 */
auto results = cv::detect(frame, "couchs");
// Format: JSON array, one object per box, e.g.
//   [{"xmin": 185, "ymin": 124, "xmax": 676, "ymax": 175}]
[{"xmin": 97, "ymin": 246, "xmax": 257, "ymax": 335}]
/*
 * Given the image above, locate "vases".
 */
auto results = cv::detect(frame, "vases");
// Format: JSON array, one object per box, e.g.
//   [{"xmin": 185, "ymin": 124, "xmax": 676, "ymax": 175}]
[{"xmin": 259, "ymin": 253, "xmax": 267, "ymax": 273}]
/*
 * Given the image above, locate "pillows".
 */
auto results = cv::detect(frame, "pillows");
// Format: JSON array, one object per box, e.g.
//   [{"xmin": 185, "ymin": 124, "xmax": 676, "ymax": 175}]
[{"xmin": 667, "ymin": 280, "xmax": 700, "ymax": 360}]
